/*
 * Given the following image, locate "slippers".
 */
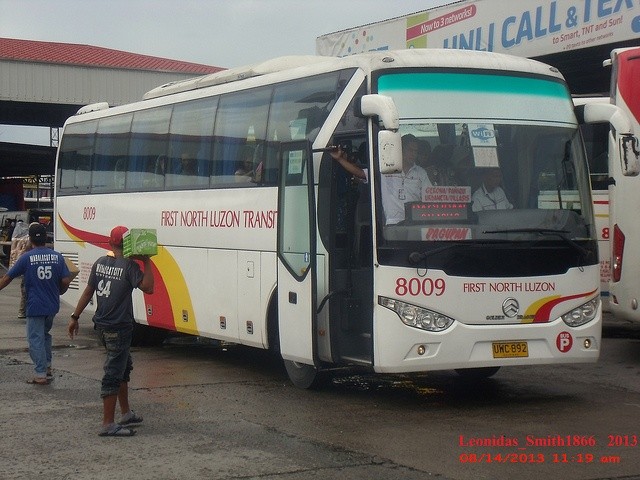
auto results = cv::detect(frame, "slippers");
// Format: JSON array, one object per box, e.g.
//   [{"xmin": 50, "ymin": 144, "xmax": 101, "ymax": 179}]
[
  {"xmin": 117, "ymin": 409, "xmax": 143, "ymax": 426},
  {"xmin": 26, "ymin": 377, "xmax": 48, "ymax": 384},
  {"xmin": 99, "ymin": 426, "xmax": 137, "ymax": 436}
]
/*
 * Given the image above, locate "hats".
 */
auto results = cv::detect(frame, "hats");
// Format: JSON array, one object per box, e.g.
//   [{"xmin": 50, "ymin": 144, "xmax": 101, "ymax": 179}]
[
  {"xmin": 28, "ymin": 224, "xmax": 46, "ymax": 238},
  {"xmin": 109, "ymin": 226, "xmax": 129, "ymax": 247}
]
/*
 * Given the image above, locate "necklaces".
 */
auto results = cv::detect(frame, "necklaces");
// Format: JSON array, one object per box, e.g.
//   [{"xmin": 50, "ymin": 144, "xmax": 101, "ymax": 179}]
[{"xmin": 396, "ymin": 170, "xmax": 412, "ymax": 186}]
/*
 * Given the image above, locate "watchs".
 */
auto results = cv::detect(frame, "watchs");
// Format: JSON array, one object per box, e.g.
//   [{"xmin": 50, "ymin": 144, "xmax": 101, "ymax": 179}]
[{"xmin": 70, "ymin": 311, "xmax": 79, "ymax": 320}]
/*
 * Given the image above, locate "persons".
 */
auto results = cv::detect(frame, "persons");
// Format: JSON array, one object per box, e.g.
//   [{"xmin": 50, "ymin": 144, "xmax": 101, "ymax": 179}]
[
  {"xmin": 180, "ymin": 151, "xmax": 197, "ymax": 185},
  {"xmin": 66, "ymin": 227, "xmax": 155, "ymax": 437},
  {"xmin": 0, "ymin": 222, "xmax": 72, "ymax": 385},
  {"xmin": 159, "ymin": 155, "xmax": 165, "ymax": 174},
  {"xmin": 329, "ymin": 133, "xmax": 433, "ymax": 225},
  {"xmin": 234, "ymin": 150, "xmax": 253, "ymax": 185},
  {"xmin": 472, "ymin": 167, "xmax": 514, "ymax": 212},
  {"xmin": 18, "ymin": 278, "xmax": 25, "ymax": 320}
]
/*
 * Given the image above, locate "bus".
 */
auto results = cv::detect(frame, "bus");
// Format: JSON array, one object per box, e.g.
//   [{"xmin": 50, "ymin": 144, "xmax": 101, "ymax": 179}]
[
  {"xmin": 53, "ymin": 49, "xmax": 602, "ymax": 391},
  {"xmin": 598, "ymin": 45, "xmax": 640, "ymax": 325},
  {"xmin": 572, "ymin": 96, "xmax": 613, "ymax": 315}
]
